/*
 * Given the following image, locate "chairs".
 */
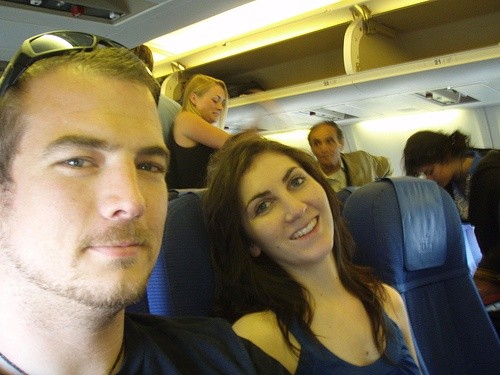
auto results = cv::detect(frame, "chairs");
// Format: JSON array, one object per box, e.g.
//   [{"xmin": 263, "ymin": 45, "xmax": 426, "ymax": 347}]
[{"xmin": 125, "ymin": 177, "xmax": 500, "ymax": 375}]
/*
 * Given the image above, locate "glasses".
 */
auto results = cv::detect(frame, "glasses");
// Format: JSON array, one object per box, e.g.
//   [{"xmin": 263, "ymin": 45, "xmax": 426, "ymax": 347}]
[{"xmin": 0, "ymin": 30, "xmax": 154, "ymax": 96}]
